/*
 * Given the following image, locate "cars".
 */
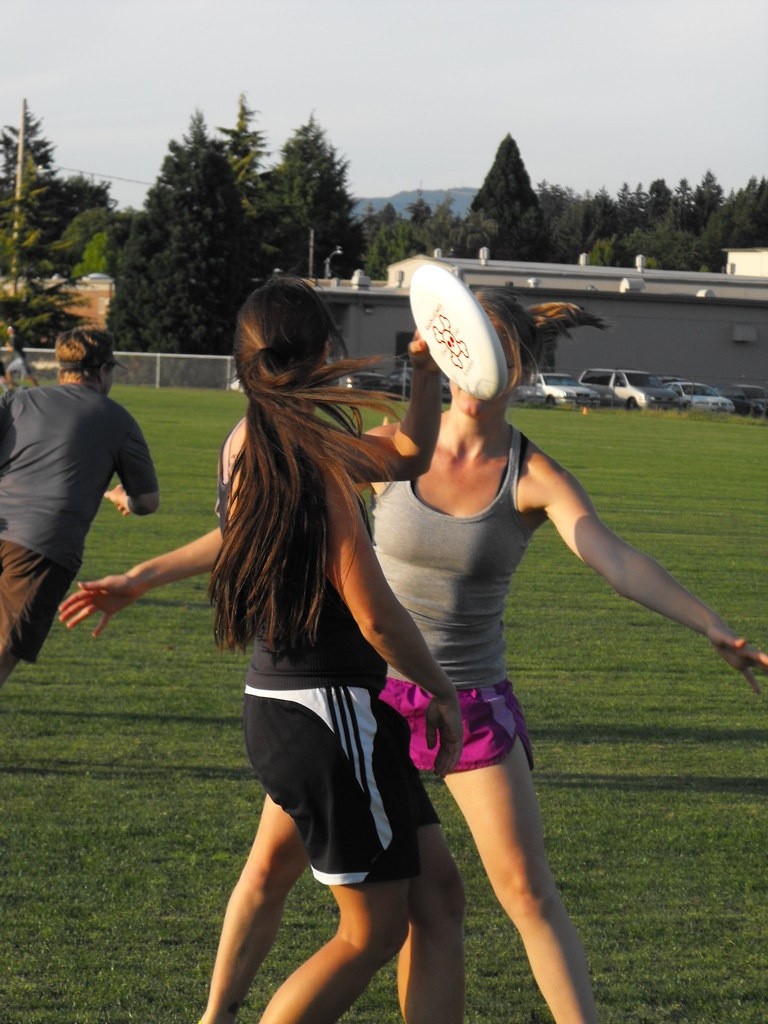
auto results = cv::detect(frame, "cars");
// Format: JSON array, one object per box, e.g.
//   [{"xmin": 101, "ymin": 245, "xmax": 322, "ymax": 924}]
[
  {"xmin": 720, "ymin": 384, "xmax": 768, "ymax": 416},
  {"xmin": 385, "ymin": 366, "xmax": 453, "ymax": 401},
  {"xmin": 510, "ymin": 372, "xmax": 603, "ymax": 409},
  {"xmin": 663, "ymin": 380, "xmax": 738, "ymax": 414}
]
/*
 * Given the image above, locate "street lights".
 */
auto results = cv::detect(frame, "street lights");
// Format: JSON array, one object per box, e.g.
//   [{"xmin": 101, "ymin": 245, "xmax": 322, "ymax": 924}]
[{"xmin": 324, "ymin": 249, "xmax": 344, "ymax": 278}]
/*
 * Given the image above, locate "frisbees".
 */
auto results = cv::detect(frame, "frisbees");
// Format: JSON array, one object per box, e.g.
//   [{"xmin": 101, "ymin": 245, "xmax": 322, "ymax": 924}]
[{"xmin": 407, "ymin": 262, "xmax": 510, "ymax": 402}]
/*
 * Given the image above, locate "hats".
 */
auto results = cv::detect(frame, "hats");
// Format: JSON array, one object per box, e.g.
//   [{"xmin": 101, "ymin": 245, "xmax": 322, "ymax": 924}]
[{"xmin": 60, "ymin": 351, "xmax": 127, "ymax": 371}]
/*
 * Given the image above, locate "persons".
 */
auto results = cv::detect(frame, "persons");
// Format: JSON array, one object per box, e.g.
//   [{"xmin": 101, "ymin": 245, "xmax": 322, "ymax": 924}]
[
  {"xmin": 0, "ymin": 319, "xmax": 160, "ymax": 684},
  {"xmin": 204, "ymin": 284, "xmax": 467, "ymax": 1023},
  {"xmin": 54, "ymin": 286, "xmax": 767, "ymax": 1023}
]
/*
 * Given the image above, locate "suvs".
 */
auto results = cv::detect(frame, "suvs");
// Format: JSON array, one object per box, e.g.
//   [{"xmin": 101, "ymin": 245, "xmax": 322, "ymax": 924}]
[
  {"xmin": 577, "ymin": 367, "xmax": 681, "ymax": 410},
  {"xmin": 325, "ymin": 361, "xmax": 394, "ymax": 394}
]
